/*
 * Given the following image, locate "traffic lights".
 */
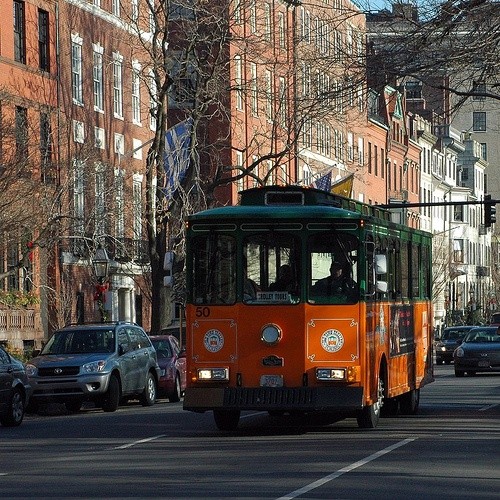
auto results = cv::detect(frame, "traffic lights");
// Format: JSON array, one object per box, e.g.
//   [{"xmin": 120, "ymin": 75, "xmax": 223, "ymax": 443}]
[{"xmin": 484, "ymin": 193, "xmax": 496, "ymax": 227}]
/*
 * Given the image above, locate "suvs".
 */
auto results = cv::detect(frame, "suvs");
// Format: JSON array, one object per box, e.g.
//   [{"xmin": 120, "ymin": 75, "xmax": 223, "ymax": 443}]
[{"xmin": 23, "ymin": 321, "xmax": 187, "ymax": 412}]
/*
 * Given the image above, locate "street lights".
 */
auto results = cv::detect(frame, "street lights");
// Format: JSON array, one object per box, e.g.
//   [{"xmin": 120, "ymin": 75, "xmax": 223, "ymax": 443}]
[{"xmin": 92, "ymin": 243, "xmax": 110, "ymax": 348}]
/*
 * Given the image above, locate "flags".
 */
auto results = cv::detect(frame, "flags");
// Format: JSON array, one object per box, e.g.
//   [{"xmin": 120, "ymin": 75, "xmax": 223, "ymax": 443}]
[
  {"xmin": 310, "ymin": 171, "xmax": 332, "ymax": 192},
  {"xmin": 164, "ymin": 118, "xmax": 193, "ymax": 200},
  {"xmin": 331, "ymin": 174, "xmax": 354, "ymax": 198}
]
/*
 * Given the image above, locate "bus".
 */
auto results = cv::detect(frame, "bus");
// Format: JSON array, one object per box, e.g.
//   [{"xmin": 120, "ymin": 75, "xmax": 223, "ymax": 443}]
[{"xmin": 182, "ymin": 185, "xmax": 435, "ymax": 430}]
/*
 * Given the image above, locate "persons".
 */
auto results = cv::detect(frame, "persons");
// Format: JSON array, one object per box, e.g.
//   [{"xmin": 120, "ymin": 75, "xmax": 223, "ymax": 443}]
[
  {"xmin": 245, "ymin": 278, "xmax": 260, "ymax": 299},
  {"xmin": 271, "ymin": 265, "xmax": 296, "ymax": 292},
  {"xmin": 314, "ymin": 261, "xmax": 356, "ymax": 289}
]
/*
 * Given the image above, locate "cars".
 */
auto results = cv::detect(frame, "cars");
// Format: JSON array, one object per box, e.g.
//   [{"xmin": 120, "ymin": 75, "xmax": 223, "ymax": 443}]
[
  {"xmin": 0, "ymin": 345, "xmax": 27, "ymax": 426},
  {"xmin": 435, "ymin": 313, "xmax": 500, "ymax": 377}
]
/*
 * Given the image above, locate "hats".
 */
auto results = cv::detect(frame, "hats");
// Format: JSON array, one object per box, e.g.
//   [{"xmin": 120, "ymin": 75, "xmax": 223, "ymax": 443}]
[{"xmin": 331, "ymin": 261, "xmax": 342, "ymax": 269}]
